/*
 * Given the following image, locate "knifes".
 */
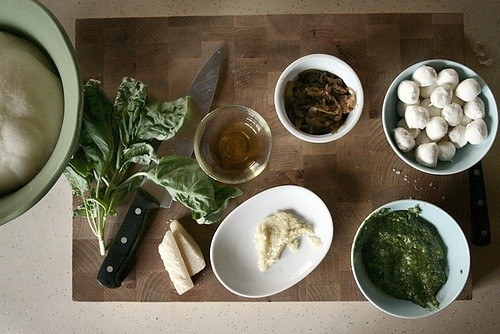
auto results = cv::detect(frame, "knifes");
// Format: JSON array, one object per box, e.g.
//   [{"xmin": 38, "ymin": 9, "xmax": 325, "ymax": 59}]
[{"xmin": 96, "ymin": 46, "xmax": 224, "ymax": 289}]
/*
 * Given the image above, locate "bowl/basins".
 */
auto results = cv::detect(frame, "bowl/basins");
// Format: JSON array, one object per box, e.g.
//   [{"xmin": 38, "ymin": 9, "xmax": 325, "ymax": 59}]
[
  {"xmin": 381, "ymin": 57, "xmax": 499, "ymax": 176},
  {"xmin": 191, "ymin": 103, "xmax": 271, "ymax": 185},
  {"xmin": 0, "ymin": 2, "xmax": 85, "ymax": 226},
  {"xmin": 350, "ymin": 198, "xmax": 472, "ymax": 320},
  {"xmin": 275, "ymin": 53, "xmax": 365, "ymax": 143}
]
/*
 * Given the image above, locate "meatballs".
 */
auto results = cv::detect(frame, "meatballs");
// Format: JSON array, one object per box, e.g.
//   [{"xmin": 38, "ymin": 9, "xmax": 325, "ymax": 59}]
[{"xmin": 394, "ymin": 65, "xmax": 488, "ymax": 168}]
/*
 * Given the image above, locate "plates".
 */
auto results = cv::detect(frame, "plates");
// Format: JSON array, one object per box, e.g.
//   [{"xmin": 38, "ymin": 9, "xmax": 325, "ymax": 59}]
[{"xmin": 209, "ymin": 185, "xmax": 334, "ymax": 299}]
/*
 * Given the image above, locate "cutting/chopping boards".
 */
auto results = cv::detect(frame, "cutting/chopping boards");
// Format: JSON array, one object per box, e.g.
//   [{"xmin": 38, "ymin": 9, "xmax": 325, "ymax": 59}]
[{"xmin": 71, "ymin": 12, "xmax": 469, "ymax": 301}]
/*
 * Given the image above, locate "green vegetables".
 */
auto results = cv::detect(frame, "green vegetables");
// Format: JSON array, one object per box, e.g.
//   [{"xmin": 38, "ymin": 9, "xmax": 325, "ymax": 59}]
[{"xmin": 64, "ymin": 76, "xmax": 243, "ymax": 258}]
